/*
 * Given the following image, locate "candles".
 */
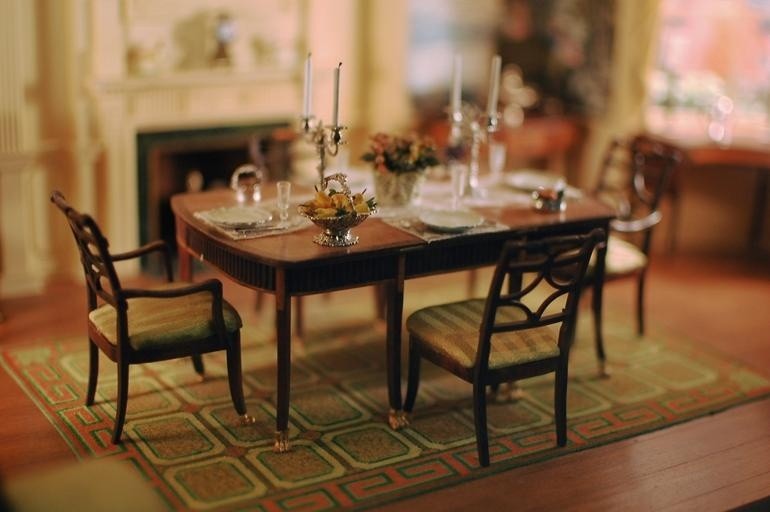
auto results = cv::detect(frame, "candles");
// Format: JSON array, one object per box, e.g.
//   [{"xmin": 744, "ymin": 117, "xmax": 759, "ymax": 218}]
[
  {"xmin": 489, "ymin": 56, "xmax": 502, "ymax": 116},
  {"xmin": 449, "ymin": 55, "xmax": 463, "ymax": 112},
  {"xmin": 331, "ymin": 62, "xmax": 343, "ymax": 128},
  {"xmin": 300, "ymin": 52, "xmax": 313, "ymax": 117}
]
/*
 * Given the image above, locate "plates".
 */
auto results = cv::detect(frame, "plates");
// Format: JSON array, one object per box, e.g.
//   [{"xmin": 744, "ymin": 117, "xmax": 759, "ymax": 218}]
[
  {"xmin": 418, "ymin": 208, "xmax": 484, "ymax": 233},
  {"xmin": 212, "ymin": 204, "xmax": 271, "ymax": 228}
]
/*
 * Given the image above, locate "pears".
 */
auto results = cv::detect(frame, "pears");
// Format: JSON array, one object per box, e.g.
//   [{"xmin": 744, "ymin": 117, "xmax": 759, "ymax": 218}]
[{"xmin": 302, "ymin": 191, "xmax": 370, "ymax": 219}]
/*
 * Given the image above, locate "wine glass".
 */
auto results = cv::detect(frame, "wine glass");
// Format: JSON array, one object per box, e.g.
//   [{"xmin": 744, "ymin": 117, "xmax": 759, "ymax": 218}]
[
  {"xmin": 298, "ymin": 200, "xmax": 379, "ymax": 247},
  {"xmin": 438, "ymin": 140, "xmax": 508, "ymax": 213},
  {"xmin": 273, "ymin": 180, "xmax": 292, "ymax": 220}
]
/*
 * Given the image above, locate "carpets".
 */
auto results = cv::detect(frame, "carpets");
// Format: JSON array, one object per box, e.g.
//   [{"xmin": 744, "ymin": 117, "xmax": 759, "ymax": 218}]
[{"xmin": 3, "ymin": 278, "xmax": 768, "ymax": 511}]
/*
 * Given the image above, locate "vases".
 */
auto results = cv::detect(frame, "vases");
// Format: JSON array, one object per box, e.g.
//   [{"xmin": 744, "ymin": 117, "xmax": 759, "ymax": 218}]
[{"xmin": 373, "ymin": 162, "xmax": 421, "ymax": 208}]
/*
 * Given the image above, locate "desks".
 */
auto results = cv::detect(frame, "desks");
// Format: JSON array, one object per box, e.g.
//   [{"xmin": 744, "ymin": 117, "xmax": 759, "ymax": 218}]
[{"xmin": 635, "ymin": 131, "xmax": 770, "ymax": 275}]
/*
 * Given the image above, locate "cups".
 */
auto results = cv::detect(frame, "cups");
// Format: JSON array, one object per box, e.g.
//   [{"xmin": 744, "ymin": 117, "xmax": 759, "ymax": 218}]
[{"xmin": 535, "ymin": 185, "xmax": 566, "ymax": 214}]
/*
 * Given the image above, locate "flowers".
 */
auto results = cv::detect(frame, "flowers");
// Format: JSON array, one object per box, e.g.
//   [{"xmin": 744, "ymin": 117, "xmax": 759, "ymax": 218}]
[{"xmin": 361, "ymin": 131, "xmax": 439, "ymax": 173}]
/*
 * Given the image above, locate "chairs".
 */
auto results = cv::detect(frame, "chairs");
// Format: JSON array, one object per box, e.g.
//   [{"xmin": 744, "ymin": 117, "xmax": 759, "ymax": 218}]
[
  {"xmin": 46, "ymin": 189, "xmax": 257, "ymax": 446},
  {"xmin": 403, "ymin": 226, "xmax": 607, "ymax": 470},
  {"xmin": 535, "ymin": 136, "xmax": 677, "ymax": 334}
]
